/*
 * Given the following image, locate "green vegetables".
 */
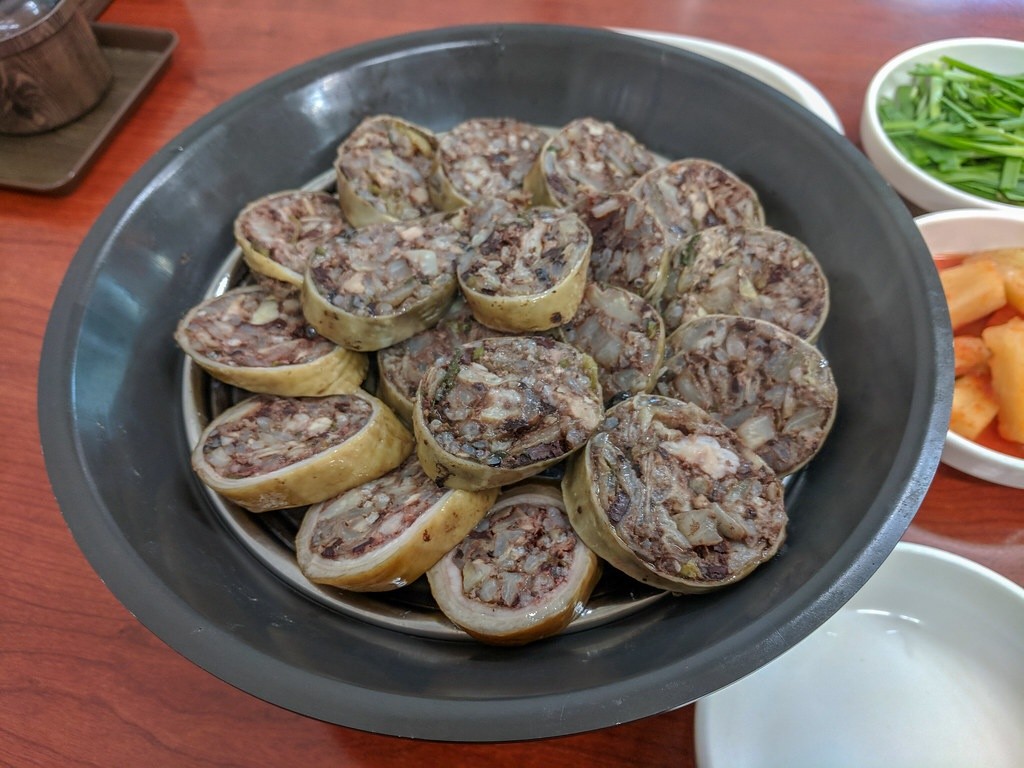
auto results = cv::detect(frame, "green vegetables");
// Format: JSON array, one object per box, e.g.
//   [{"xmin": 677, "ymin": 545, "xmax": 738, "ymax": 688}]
[{"xmin": 878, "ymin": 54, "xmax": 1024, "ymax": 209}]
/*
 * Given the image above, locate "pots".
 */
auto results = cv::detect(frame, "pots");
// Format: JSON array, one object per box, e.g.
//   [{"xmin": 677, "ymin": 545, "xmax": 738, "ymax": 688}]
[
  {"xmin": 0, "ymin": 0, "xmax": 115, "ymax": 136},
  {"xmin": 35, "ymin": 21, "xmax": 956, "ymax": 743}
]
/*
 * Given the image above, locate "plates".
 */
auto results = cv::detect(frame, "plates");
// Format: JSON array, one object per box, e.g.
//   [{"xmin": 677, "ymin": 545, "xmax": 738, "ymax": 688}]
[{"xmin": 4, "ymin": 26, "xmax": 178, "ymax": 192}]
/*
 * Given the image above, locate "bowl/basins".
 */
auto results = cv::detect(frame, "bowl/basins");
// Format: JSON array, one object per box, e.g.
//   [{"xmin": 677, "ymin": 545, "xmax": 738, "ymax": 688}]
[
  {"xmin": 912, "ymin": 207, "xmax": 1024, "ymax": 490},
  {"xmin": 859, "ymin": 37, "xmax": 1024, "ymax": 212},
  {"xmin": 692, "ymin": 541, "xmax": 1024, "ymax": 768}
]
